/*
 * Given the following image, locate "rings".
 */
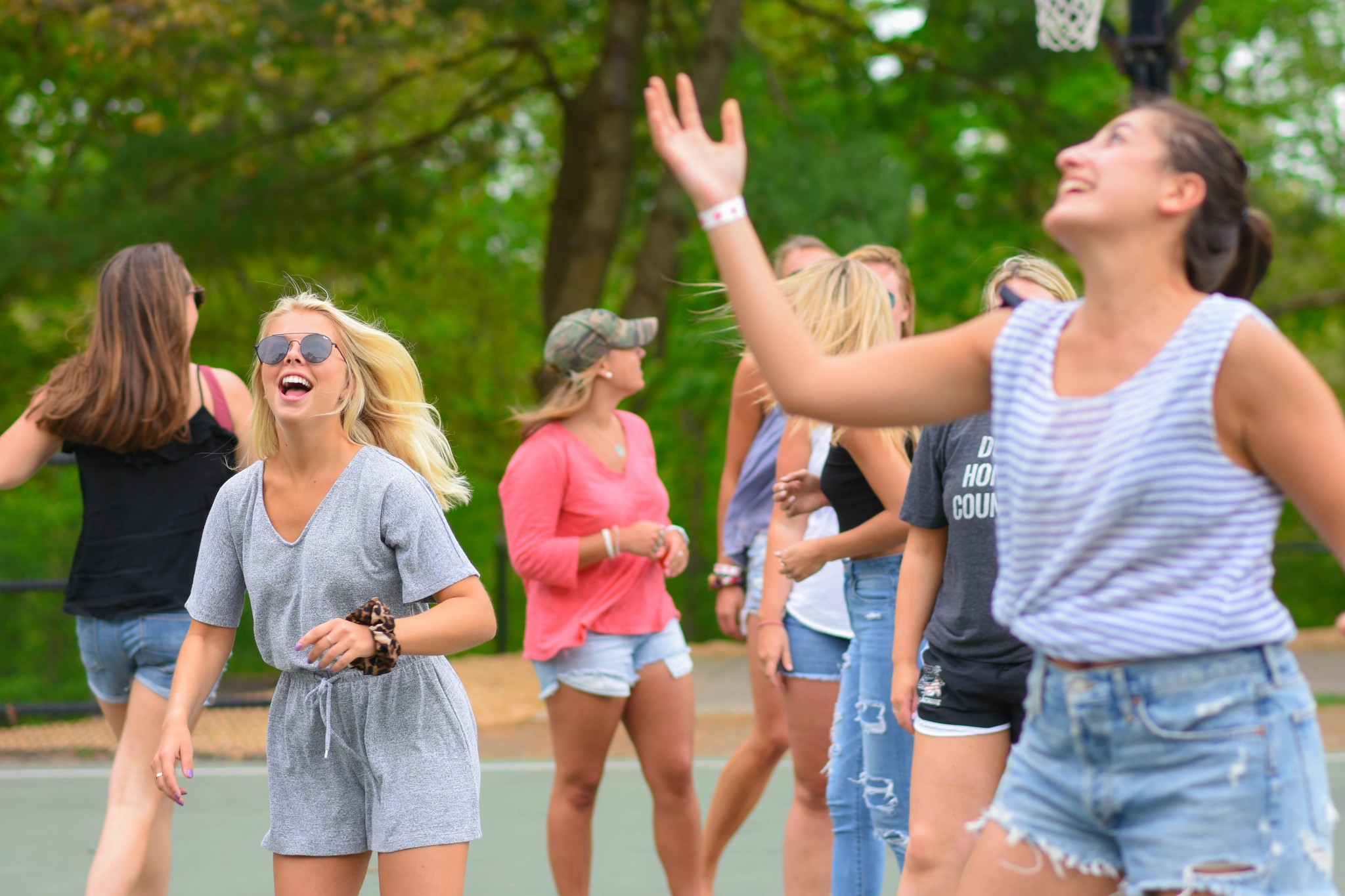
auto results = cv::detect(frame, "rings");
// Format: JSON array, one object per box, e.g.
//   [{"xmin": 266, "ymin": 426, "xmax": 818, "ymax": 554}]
[
  {"xmin": 677, "ymin": 551, "xmax": 683, "ymax": 555},
  {"xmin": 325, "ymin": 635, "xmax": 334, "ymax": 646},
  {"xmin": 156, "ymin": 772, "xmax": 163, "ymax": 779}
]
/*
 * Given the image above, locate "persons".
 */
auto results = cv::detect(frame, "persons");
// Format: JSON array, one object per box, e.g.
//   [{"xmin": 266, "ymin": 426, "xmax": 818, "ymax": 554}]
[
  {"xmin": 643, "ymin": 72, "xmax": 1345, "ymax": 896},
  {"xmin": 0, "ymin": 242, "xmax": 261, "ymax": 896},
  {"xmin": 700, "ymin": 233, "xmax": 915, "ymax": 896},
  {"xmin": 653, "ymin": 257, "xmax": 922, "ymax": 896},
  {"xmin": 886, "ymin": 253, "xmax": 1078, "ymax": 896},
  {"xmin": 498, "ymin": 308, "xmax": 703, "ymax": 896},
  {"xmin": 150, "ymin": 270, "xmax": 497, "ymax": 896}
]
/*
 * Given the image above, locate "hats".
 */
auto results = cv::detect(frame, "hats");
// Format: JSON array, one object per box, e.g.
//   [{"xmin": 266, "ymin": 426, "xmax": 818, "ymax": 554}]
[{"xmin": 543, "ymin": 308, "xmax": 658, "ymax": 376}]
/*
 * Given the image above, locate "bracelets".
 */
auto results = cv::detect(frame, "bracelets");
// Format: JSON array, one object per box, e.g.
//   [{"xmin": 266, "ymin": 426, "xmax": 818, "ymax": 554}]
[
  {"xmin": 664, "ymin": 524, "xmax": 690, "ymax": 545},
  {"xmin": 344, "ymin": 597, "xmax": 401, "ymax": 676},
  {"xmin": 697, "ymin": 193, "xmax": 747, "ymax": 229},
  {"xmin": 712, "ymin": 563, "xmax": 745, "ymax": 577},
  {"xmin": 601, "ymin": 528, "xmax": 615, "ymax": 558},
  {"xmin": 707, "ymin": 574, "xmax": 745, "ymax": 589},
  {"xmin": 612, "ymin": 523, "xmax": 622, "ymax": 555},
  {"xmin": 759, "ymin": 620, "xmax": 786, "ymax": 626}
]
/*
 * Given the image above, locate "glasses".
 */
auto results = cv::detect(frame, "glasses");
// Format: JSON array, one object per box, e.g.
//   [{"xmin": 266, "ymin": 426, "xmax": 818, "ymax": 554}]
[
  {"xmin": 254, "ymin": 332, "xmax": 348, "ymax": 365},
  {"xmin": 186, "ymin": 285, "xmax": 205, "ymax": 309},
  {"xmin": 888, "ymin": 291, "xmax": 906, "ymax": 308}
]
